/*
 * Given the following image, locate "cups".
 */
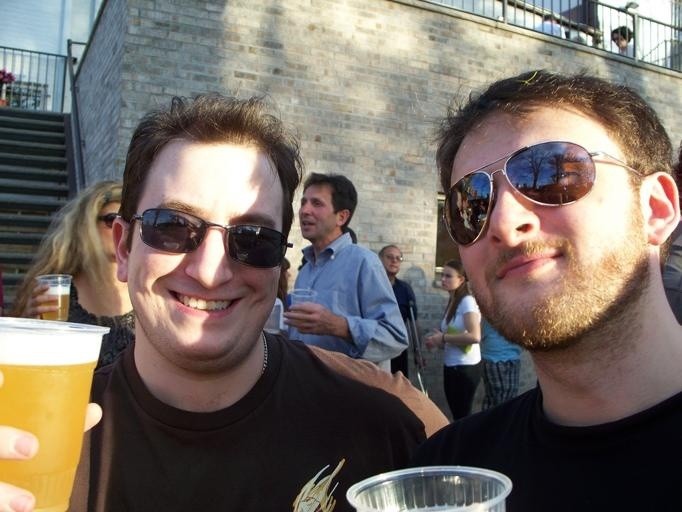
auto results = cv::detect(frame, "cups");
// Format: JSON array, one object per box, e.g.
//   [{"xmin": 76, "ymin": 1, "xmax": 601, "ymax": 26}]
[
  {"xmin": 0, "ymin": 316, "xmax": 111, "ymax": 512},
  {"xmin": 35, "ymin": 274, "xmax": 72, "ymax": 322},
  {"xmin": 290, "ymin": 288, "xmax": 318, "ymax": 323}
]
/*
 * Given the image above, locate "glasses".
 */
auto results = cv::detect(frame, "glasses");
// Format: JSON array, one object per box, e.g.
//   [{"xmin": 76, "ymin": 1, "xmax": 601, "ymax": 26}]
[
  {"xmin": 96, "ymin": 213, "xmax": 117, "ymax": 228},
  {"xmin": 384, "ymin": 254, "xmax": 404, "ymax": 262},
  {"xmin": 132, "ymin": 209, "xmax": 292, "ymax": 266},
  {"xmin": 440, "ymin": 143, "xmax": 645, "ymax": 245}
]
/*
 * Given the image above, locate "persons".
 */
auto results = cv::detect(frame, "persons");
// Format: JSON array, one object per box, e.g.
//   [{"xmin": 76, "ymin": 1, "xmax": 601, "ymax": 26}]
[
  {"xmin": 658, "ymin": 145, "xmax": 682, "ymax": 325},
  {"xmin": 532, "ymin": 12, "xmax": 568, "ymax": 41},
  {"xmin": 480, "ymin": 315, "xmax": 524, "ymax": 413},
  {"xmin": 283, "ymin": 168, "xmax": 411, "ymax": 370},
  {"xmin": 276, "ymin": 258, "xmax": 292, "ymax": 314},
  {"xmin": 425, "ymin": 256, "xmax": 485, "ymax": 421},
  {"xmin": 0, "ymin": 89, "xmax": 429, "ymax": 512},
  {"xmin": 377, "ymin": 241, "xmax": 425, "ymax": 386},
  {"xmin": 609, "ymin": 25, "xmax": 644, "ymax": 63},
  {"xmin": 12, "ymin": 179, "xmax": 136, "ymax": 375},
  {"xmin": 391, "ymin": 64, "xmax": 681, "ymax": 512}
]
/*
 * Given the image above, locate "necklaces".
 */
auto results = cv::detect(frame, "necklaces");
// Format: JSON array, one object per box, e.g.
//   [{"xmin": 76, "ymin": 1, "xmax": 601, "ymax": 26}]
[{"xmin": 258, "ymin": 329, "xmax": 271, "ymax": 377}]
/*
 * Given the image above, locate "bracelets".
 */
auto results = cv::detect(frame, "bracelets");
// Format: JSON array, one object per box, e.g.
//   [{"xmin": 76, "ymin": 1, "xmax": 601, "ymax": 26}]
[{"xmin": 441, "ymin": 332, "xmax": 450, "ymax": 345}]
